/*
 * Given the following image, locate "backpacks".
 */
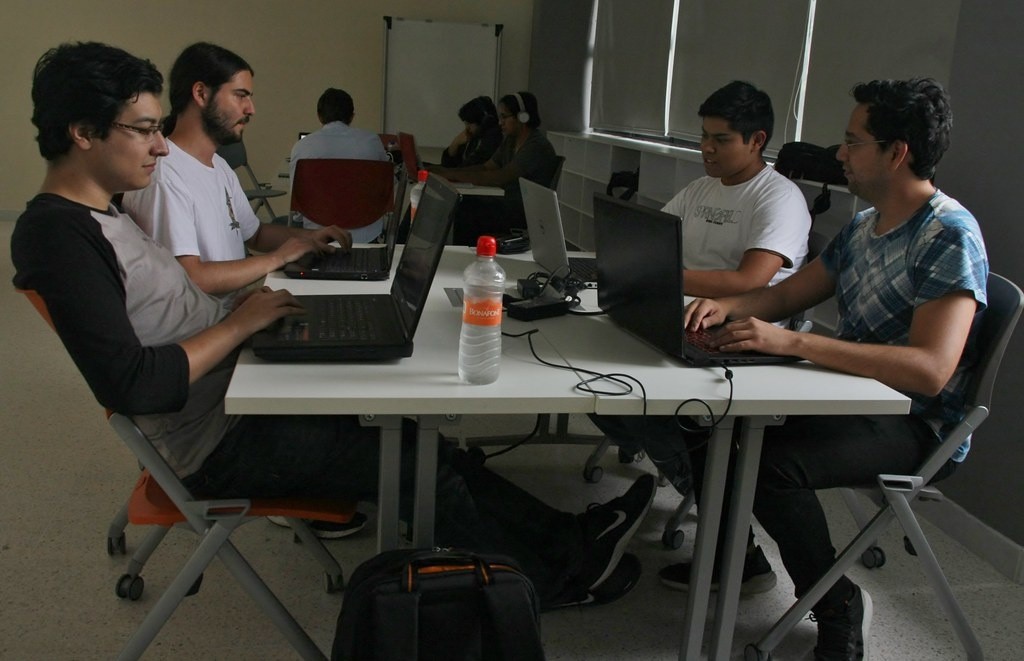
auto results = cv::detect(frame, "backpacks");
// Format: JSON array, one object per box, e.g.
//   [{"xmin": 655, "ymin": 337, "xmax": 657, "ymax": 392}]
[{"xmin": 330, "ymin": 546, "xmax": 545, "ymax": 661}]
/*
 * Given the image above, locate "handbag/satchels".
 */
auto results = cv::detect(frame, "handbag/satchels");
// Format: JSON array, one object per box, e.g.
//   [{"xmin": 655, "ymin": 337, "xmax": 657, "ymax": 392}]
[{"xmin": 775, "ymin": 142, "xmax": 848, "ymax": 184}]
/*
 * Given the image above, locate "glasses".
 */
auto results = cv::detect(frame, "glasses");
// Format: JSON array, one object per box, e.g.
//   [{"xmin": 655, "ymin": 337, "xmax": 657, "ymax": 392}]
[
  {"xmin": 111, "ymin": 122, "xmax": 163, "ymax": 142},
  {"xmin": 498, "ymin": 114, "xmax": 514, "ymax": 119},
  {"xmin": 840, "ymin": 141, "xmax": 887, "ymax": 149}
]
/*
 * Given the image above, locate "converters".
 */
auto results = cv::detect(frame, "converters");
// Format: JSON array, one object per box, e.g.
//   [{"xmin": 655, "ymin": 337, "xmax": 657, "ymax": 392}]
[{"xmin": 507, "ymin": 296, "xmax": 568, "ymax": 322}]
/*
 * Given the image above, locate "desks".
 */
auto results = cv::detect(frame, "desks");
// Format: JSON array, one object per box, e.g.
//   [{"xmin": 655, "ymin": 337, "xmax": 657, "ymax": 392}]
[{"xmin": 225, "ymin": 184, "xmax": 913, "ymax": 661}]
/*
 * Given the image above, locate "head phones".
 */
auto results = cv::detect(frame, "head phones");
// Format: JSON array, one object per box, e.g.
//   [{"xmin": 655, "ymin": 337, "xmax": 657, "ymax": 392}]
[
  {"xmin": 514, "ymin": 93, "xmax": 530, "ymax": 124},
  {"xmin": 481, "ymin": 109, "xmax": 495, "ymax": 128}
]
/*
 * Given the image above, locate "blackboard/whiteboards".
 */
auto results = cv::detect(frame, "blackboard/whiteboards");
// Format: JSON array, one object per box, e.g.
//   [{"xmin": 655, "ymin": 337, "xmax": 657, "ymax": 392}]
[{"xmin": 381, "ymin": 16, "xmax": 506, "ymax": 149}]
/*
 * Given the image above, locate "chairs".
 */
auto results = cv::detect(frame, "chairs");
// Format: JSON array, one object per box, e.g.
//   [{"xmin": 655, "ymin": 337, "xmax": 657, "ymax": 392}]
[
  {"xmin": 500, "ymin": 156, "xmax": 566, "ymax": 230},
  {"xmin": 660, "ymin": 267, "xmax": 1024, "ymax": 661},
  {"xmin": 588, "ymin": 178, "xmax": 863, "ymax": 531},
  {"xmin": 290, "ymin": 159, "xmax": 394, "ymax": 245},
  {"xmin": 161, "ymin": 113, "xmax": 288, "ymax": 225},
  {"xmin": 10, "ymin": 271, "xmax": 359, "ymax": 661}
]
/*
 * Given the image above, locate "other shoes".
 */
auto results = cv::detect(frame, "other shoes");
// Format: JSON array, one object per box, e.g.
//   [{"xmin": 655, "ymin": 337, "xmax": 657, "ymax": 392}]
[{"xmin": 266, "ymin": 512, "xmax": 368, "ymax": 538}]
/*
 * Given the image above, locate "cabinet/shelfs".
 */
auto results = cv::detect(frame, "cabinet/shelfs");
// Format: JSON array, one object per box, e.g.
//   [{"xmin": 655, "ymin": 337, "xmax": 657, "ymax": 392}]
[{"xmin": 543, "ymin": 132, "xmax": 714, "ymax": 251}]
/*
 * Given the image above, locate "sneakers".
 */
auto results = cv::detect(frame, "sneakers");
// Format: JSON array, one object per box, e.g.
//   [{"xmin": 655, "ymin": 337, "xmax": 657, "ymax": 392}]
[
  {"xmin": 806, "ymin": 583, "xmax": 872, "ymax": 661},
  {"xmin": 564, "ymin": 474, "xmax": 656, "ymax": 590},
  {"xmin": 546, "ymin": 553, "xmax": 641, "ymax": 610},
  {"xmin": 662, "ymin": 546, "xmax": 776, "ymax": 598}
]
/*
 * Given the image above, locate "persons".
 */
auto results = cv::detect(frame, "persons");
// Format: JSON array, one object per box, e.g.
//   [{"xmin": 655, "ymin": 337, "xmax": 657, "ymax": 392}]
[
  {"xmin": 657, "ymin": 75, "xmax": 990, "ymax": 661},
  {"xmin": 422, "ymin": 92, "xmax": 556, "ymax": 248},
  {"xmin": 288, "ymin": 87, "xmax": 394, "ymax": 243},
  {"xmin": 585, "ymin": 82, "xmax": 812, "ymax": 496},
  {"xmin": 11, "ymin": 41, "xmax": 656, "ymax": 611},
  {"xmin": 121, "ymin": 42, "xmax": 367, "ymax": 541}
]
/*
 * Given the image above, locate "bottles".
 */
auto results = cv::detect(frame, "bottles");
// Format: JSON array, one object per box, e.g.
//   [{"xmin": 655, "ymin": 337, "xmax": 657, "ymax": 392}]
[
  {"xmin": 457, "ymin": 236, "xmax": 507, "ymax": 387},
  {"xmin": 409, "ymin": 170, "xmax": 428, "ymax": 227}
]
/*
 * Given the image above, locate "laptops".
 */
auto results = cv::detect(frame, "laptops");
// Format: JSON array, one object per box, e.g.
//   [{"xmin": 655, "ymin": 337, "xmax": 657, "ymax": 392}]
[
  {"xmin": 283, "ymin": 161, "xmax": 409, "ymax": 281},
  {"xmin": 253, "ymin": 173, "xmax": 462, "ymax": 367},
  {"xmin": 379, "ymin": 132, "xmax": 473, "ymax": 189},
  {"xmin": 518, "ymin": 177, "xmax": 598, "ymax": 288},
  {"xmin": 299, "ymin": 132, "xmax": 310, "ymax": 141},
  {"xmin": 592, "ymin": 192, "xmax": 805, "ymax": 367}
]
